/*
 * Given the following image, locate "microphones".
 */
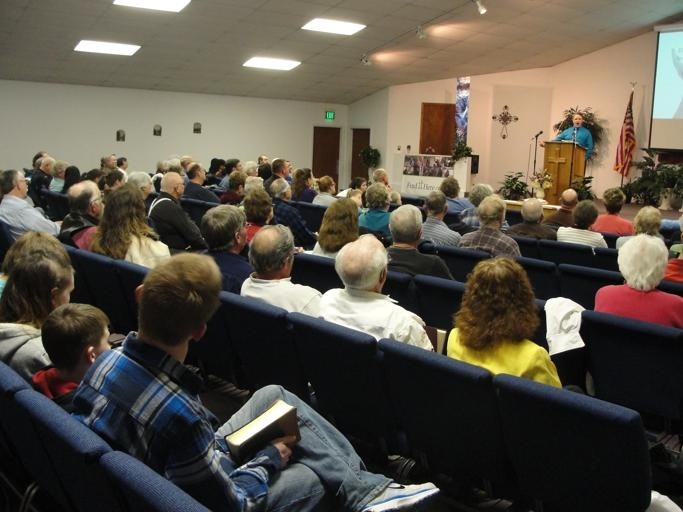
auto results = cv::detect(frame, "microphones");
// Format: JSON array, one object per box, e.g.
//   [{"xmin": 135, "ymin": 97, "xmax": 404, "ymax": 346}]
[
  {"xmin": 573, "ymin": 128, "xmax": 575, "ymax": 141},
  {"xmin": 532, "ymin": 130, "xmax": 543, "ymax": 139}
]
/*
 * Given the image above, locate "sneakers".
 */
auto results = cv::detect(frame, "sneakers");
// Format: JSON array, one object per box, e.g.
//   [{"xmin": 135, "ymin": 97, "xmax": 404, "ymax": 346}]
[{"xmin": 361, "ymin": 481, "xmax": 440, "ymax": 512}]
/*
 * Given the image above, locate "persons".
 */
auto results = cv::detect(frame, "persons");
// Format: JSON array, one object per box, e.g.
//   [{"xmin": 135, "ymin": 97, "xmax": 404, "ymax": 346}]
[
  {"xmin": 0, "ymin": 151, "xmax": 683, "ymax": 512},
  {"xmin": 538, "ymin": 113, "xmax": 593, "ymax": 163}
]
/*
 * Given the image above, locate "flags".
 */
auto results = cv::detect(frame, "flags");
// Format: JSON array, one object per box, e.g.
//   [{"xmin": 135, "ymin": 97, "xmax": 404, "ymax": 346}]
[{"xmin": 610, "ymin": 89, "xmax": 635, "ymax": 178}]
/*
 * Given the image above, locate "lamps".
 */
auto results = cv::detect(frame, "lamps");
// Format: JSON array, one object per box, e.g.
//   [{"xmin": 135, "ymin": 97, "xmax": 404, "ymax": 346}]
[{"xmin": 358, "ymin": 0, "xmax": 488, "ymax": 67}]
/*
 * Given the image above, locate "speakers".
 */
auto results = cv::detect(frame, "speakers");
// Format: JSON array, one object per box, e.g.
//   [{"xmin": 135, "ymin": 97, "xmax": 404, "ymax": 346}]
[{"xmin": 468, "ymin": 155, "xmax": 478, "ymax": 173}]
